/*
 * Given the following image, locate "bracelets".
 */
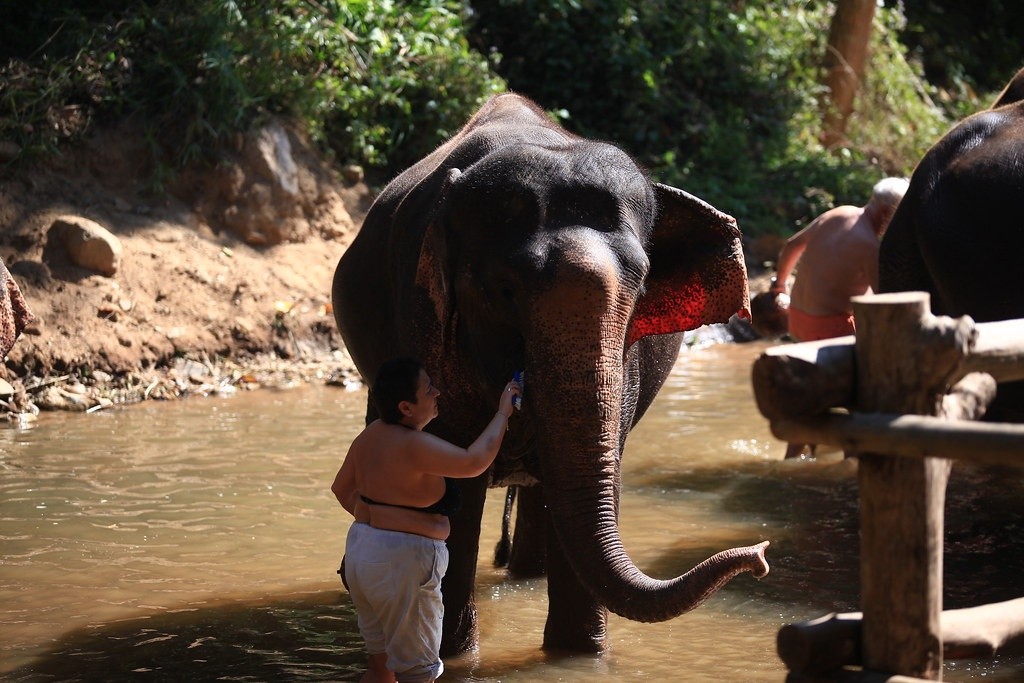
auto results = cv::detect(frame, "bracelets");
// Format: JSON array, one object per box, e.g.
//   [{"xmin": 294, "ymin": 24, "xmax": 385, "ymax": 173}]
[
  {"xmin": 494, "ymin": 413, "xmax": 509, "ymax": 431},
  {"xmin": 771, "ymin": 277, "xmax": 785, "ymax": 288}
]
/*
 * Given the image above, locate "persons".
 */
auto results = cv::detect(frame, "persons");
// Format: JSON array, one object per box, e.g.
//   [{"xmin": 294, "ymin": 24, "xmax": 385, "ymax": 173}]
[
  {"xmin": 331, "ymin": 366, "xmax": 523, "ymax": 683},
  {"xmin": 769, "ymin": 177, "xmax": 910, "ymax": 460}
]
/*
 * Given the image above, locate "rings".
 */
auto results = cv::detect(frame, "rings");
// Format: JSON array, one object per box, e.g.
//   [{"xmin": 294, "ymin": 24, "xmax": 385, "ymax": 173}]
[{"xmin": 507, "ymin": 388, "xmax": 511, "ymax": 392}]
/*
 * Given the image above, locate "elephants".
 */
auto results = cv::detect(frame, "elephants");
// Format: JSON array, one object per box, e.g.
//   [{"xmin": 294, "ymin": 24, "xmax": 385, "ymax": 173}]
[
  {"xmin": 330, "ymin": 89, "xmax": 773, "ymax": 662},
  {"xmin": 871, "ymin": 62, "xmax": 1024, "ymax": 479}
]
